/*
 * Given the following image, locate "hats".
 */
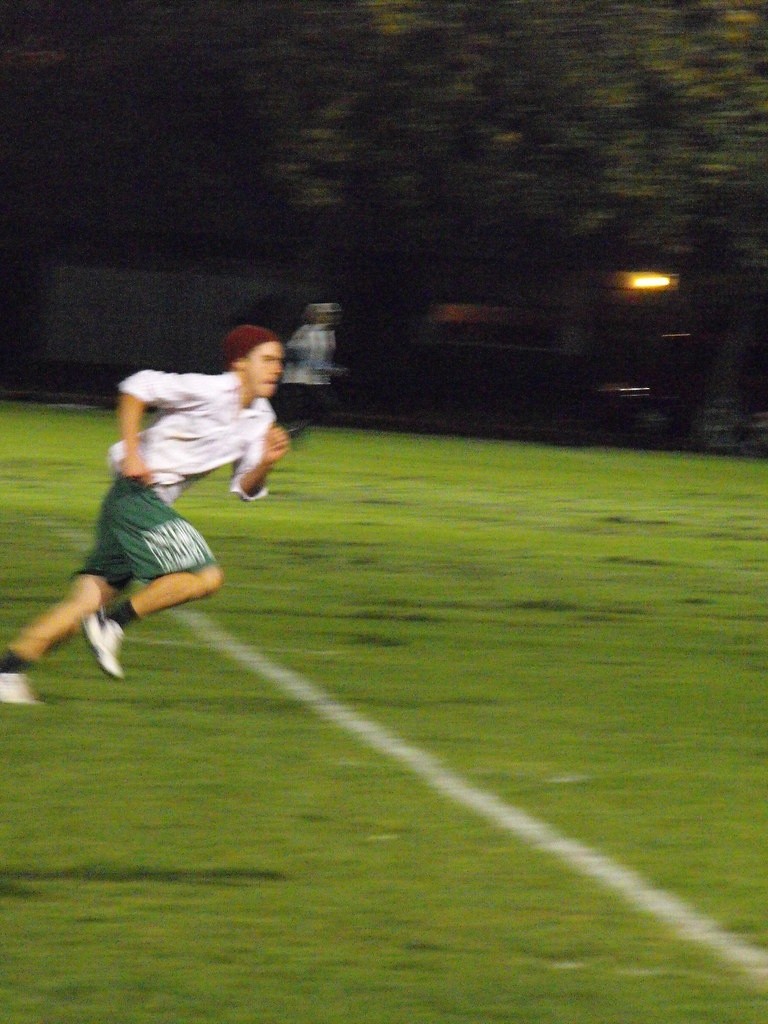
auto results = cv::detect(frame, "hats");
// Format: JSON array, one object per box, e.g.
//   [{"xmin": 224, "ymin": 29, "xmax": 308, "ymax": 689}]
[{"xmin": 223, "ymin": 325, "xmax": 279, "ymax": 365}]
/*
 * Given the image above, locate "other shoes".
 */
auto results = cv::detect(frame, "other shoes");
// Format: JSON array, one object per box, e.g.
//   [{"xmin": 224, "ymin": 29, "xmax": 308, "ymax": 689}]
[
  {"xmin": 82, "ymin": 610, "xmax": 126, "ymax": 680},
  {"xmin": 0, "ymin": 673, "xmax": 33, "ymax": 704}
]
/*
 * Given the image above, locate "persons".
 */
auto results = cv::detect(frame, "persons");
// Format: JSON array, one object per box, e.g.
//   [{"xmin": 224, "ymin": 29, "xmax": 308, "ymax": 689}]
[
  {"xmin": 0, "ymin": 325, "xmax": 291, "ymax": 705},
  {"xmin": 278, "ymin": 304, "xmax": 337, "ymax": 438}
]
